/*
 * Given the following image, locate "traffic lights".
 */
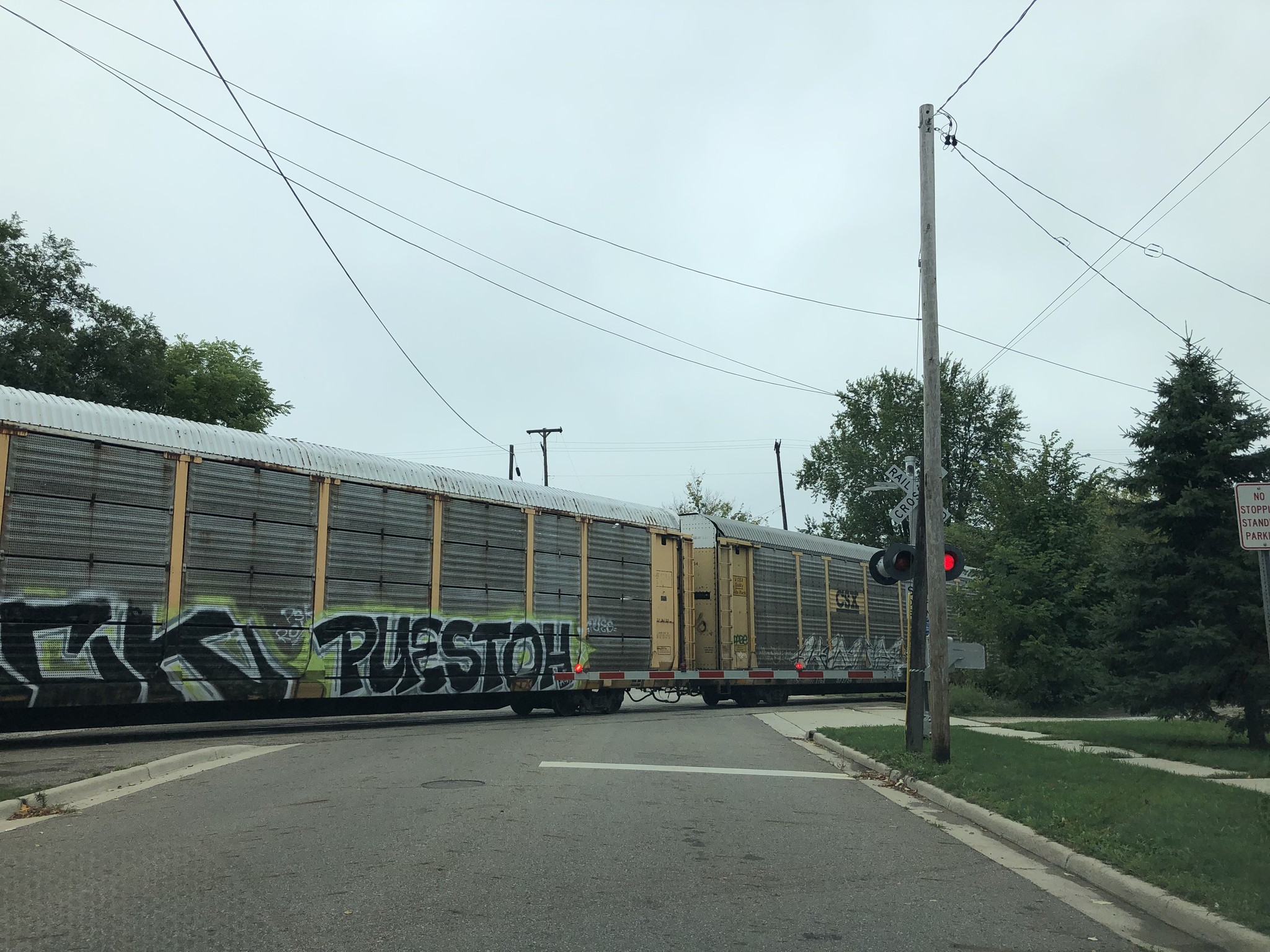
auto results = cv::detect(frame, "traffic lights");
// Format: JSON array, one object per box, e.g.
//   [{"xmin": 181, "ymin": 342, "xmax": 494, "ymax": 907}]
[
  {"xmin": 868, "ymin": 540, "xmax": 915, "ymax": 586},
  {"xmin": 943, "ymin": 543, "xmax": 966, "ymax": 582}
]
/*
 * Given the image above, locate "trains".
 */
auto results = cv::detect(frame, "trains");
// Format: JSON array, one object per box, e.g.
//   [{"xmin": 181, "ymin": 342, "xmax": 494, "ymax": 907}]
[{"xmin": 0, "ymin": 384, "xmax": 998, "ymax": 742}]
